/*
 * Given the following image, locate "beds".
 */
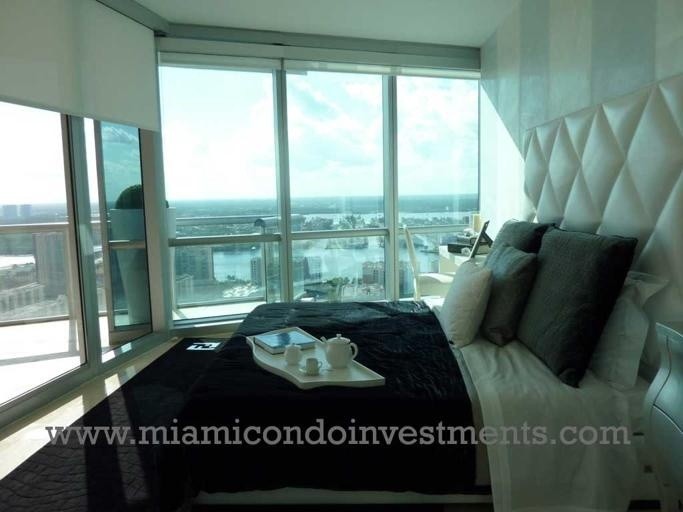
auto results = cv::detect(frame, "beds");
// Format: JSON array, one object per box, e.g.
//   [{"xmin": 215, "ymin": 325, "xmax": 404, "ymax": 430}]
[{"xmin": 173, "ymin": 73, "xmax": 683, "ymax": 505}]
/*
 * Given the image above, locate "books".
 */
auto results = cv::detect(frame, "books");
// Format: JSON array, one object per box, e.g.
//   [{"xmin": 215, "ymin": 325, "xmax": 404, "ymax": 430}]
[{"xmin": 253, "ymin": 329, "xmax": 315, "ymax": 355}]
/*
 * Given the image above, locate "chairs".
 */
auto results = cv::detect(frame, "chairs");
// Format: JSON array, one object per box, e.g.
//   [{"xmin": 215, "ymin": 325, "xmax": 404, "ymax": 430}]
[{"xmin": 405, "ymin": 225, "xmax": 454, "ymax": 302}]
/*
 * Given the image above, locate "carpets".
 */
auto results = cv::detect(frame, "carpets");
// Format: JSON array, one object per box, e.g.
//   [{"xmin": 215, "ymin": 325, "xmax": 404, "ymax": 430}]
[{"xmin": 0, "ymin": 336, "xmax": 682, "ymax": 511}]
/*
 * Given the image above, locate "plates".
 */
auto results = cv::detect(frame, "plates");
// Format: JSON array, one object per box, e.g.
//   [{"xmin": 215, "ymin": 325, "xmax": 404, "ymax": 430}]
[{"xmin": 299, "ymin": 366, "xmax": 322, "ymax": 375}]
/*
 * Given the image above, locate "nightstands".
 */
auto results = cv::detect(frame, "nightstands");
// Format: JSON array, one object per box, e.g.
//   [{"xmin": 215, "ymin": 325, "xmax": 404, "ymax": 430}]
[{"xmin": 438, "ymin": 244, "xmax": 494, "ymax": 279}]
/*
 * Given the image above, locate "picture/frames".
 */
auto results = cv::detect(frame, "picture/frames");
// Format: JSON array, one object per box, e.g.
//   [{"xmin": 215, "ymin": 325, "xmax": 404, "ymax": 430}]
[{"xmin": 469, "ymin": 221, "xmax": 489, "ymax": 259}]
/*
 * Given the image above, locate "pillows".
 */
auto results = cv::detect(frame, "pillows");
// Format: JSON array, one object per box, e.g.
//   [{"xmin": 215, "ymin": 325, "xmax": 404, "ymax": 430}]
[{"xmin": 438, "ymin": 216, "xmax": 668, "ymax": 392}]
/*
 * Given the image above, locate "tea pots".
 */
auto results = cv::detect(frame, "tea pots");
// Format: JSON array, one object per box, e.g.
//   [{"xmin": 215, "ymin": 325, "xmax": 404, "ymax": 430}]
[{"xmin": 316, "ymin": 333, "xmax": 359, "ymax": 370}]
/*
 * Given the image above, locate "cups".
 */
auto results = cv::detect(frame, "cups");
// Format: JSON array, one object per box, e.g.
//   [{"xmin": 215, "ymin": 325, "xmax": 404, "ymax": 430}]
[
  {"xmin": 284, "ymin": 342, "xmax": 303, "ymax": 364},
  {"xmin": 306, "ymin": 358, "xmax": 322, "ymax": 370}
]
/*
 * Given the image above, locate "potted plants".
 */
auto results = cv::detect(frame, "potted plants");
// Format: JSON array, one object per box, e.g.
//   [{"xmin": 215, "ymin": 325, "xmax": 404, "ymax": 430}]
[{"xmin": 109, "ymin": 184, "xmax": 176, "ymax": 325}]
